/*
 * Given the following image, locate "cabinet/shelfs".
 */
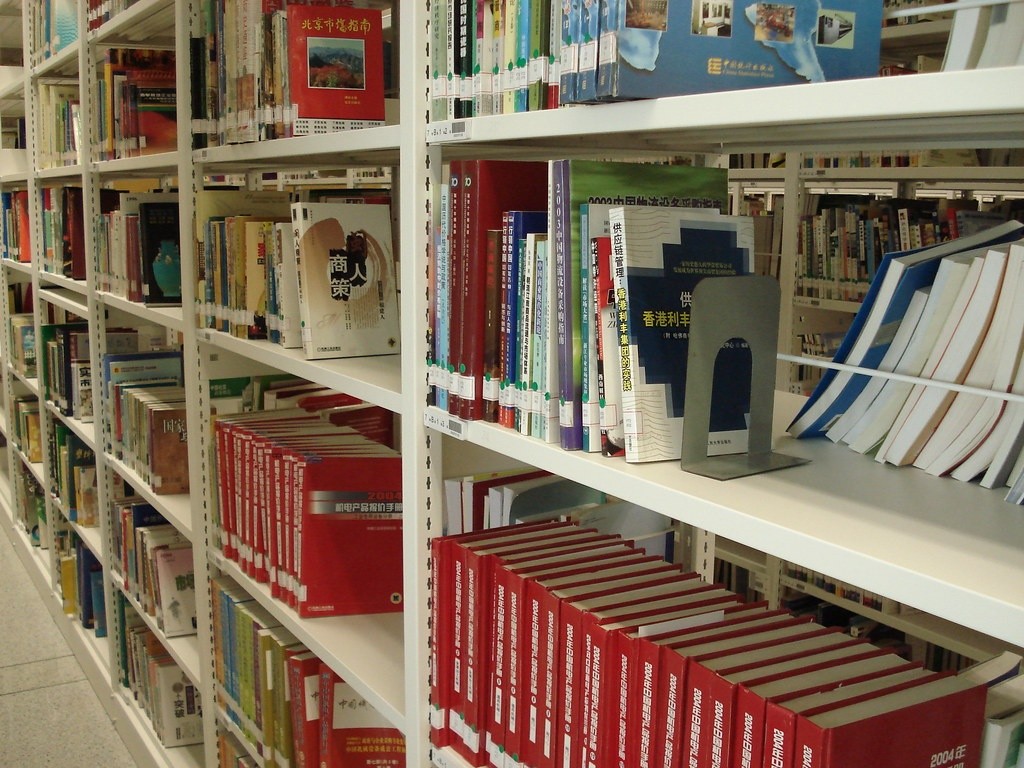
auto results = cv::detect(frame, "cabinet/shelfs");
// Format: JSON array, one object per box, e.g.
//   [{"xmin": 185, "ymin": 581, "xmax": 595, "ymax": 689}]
[{"xmin": 0, "ymin": 0, "xmax": 1024, "ymax": 768}]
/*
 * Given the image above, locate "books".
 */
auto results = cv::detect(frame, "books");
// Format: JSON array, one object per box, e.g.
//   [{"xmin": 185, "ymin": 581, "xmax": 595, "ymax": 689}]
[
  {"xmin": 0, "ymin": 0, "xmax": 406, "ymax": 768},
  {"xmin": 427, "ymin": 0, "xmax": 1024, "ymax": 768}
]
